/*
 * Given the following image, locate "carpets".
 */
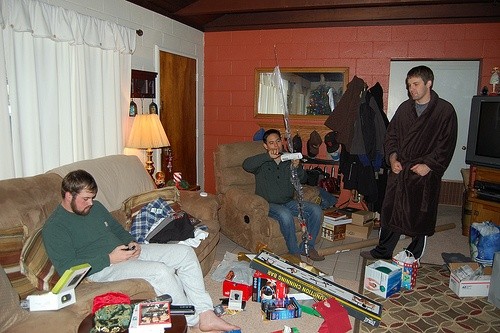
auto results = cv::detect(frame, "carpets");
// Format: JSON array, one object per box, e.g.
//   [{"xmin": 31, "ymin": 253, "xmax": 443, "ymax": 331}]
[{"xmin": 354, "ymin": 258, "xmax": 500, "ymax": 333}]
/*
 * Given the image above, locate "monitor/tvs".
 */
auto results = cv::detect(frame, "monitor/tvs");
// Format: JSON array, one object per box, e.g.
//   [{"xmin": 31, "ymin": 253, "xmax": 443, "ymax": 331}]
[{"xmin": 464, "ymin": 96, "xmax": 500, "ymax": 169}]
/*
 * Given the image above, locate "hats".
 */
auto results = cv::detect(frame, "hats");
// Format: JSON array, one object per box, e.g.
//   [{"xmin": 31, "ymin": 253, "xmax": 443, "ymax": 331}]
[
  {"xmin": 293, "ymin": 134, "xmax": 302, "ymax": 154},
  {"xmin": 309, "ymin": 130, "xmax": 323, "ymax": 154},
  {"xmin": 325, "ymin": 132, "xmax": 339, "ymax": 153}
]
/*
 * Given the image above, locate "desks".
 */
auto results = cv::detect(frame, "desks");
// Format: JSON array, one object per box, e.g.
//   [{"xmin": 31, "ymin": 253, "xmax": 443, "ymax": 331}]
[{"xmin": 78, "ymin": 299, "xmax": 187, "ymax": 333}]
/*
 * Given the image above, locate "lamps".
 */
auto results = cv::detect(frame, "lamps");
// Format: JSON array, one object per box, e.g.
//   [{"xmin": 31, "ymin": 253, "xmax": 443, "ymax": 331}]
[{"xmin": 126, "ymin": 114, "xmax": 172, "ymax": 174}]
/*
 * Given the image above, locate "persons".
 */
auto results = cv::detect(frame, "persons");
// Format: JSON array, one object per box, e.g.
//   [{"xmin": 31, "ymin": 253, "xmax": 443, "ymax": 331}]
[
  {"xmin": 42, "ymin": 169, "xmax": 241, "ymax": 331},
  {"xmin": 241, "ymin": 129, "xmax": 325, "ymax": 262},
  {"xmin": 360, "ymin": 65, "xmax": 459, "ymax": 268}
]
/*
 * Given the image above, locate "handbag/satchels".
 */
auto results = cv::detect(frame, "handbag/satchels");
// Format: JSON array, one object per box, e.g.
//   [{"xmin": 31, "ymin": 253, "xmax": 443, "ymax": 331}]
[
  {"xmin": 320, "ymin": 178, "xmax": 338, "ymax": 193},
  {"xmin": 470, "ymin": 220, "xmax": 500, "ymax": 266},
  {"xmin": 392, "ymin": 250, "xmax": 417, "ymax": 290}
]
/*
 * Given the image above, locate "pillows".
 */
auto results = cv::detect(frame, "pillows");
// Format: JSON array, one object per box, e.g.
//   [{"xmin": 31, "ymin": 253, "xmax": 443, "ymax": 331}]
[
  {"xmin": 0, "ymin": 226, "xmax": 57, "ymax": 333},
  {"xmin": 107, "ymin": 185, "xmax": 201, "ymax": 244},
  {"xmin": 211, "ymin": 250, "xmax": 257, "ymax": 285}
]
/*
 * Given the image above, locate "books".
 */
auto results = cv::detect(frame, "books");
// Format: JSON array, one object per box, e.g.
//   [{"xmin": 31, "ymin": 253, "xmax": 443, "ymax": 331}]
[{"xmin": 128, "ymin": 302, "xmax": 172, "ymax": 333}]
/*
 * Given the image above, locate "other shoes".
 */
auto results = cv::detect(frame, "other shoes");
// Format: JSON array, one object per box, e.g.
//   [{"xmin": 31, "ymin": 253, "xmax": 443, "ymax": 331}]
[
  {"xmin": 301, "ymin": 248, "xmax": 325, "ymax": 261},
  {"xmin": 360, "ymin": 251, "xmax": 392, "ymax": 262}
]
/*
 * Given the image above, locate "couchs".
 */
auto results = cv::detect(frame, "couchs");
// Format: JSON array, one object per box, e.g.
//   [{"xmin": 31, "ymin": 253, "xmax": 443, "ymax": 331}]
[
  {"xmin": 214, "ymin": 141, "xmax": 323, "ymax": 255},
  {"xmin": 0, "ymin": 154, "xmax": 220, "ymax": 333}
]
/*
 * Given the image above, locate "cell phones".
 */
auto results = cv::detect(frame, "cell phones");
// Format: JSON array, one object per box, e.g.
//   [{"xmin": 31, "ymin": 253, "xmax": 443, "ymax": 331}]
[{"xmin": 131, "ymin": 246, "xmax": 136, "ymax": 250}]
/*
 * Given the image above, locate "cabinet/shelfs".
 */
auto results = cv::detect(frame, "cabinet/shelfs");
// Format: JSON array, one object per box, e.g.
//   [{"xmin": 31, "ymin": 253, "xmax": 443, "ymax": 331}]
[
  {"xmin": 461, "ymin": 165, "xmax": 500, "ymax": 235},
  {"xmin": 297, "ymin": 159, "xmax": 345, "ymax": 198}
]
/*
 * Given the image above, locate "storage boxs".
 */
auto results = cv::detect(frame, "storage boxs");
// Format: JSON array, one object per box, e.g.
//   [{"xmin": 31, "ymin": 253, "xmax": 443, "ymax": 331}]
[
  {"xmin": 223, "ymin": 280, "xmax": 253, "ymax": 301},
  {"xmin": 321, "ymin": 209, "xmax": 375, "ymax": 241},
  {"xmin": 448, "ymin": 262, "xmax": 491, "ymax": 297},
  {"xmin": 252, "ymin": 250, "xmax": 401, "ymax": 328},
  {"xmin": 30, "ymin": 263, "xmax": 92, "ymax": 312}
]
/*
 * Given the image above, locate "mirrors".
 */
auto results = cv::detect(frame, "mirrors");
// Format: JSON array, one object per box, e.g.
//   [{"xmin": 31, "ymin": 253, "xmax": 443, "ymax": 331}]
[{"xmin": 253, "ymin": 66, "xmax": 349, "ymax": 119}]
